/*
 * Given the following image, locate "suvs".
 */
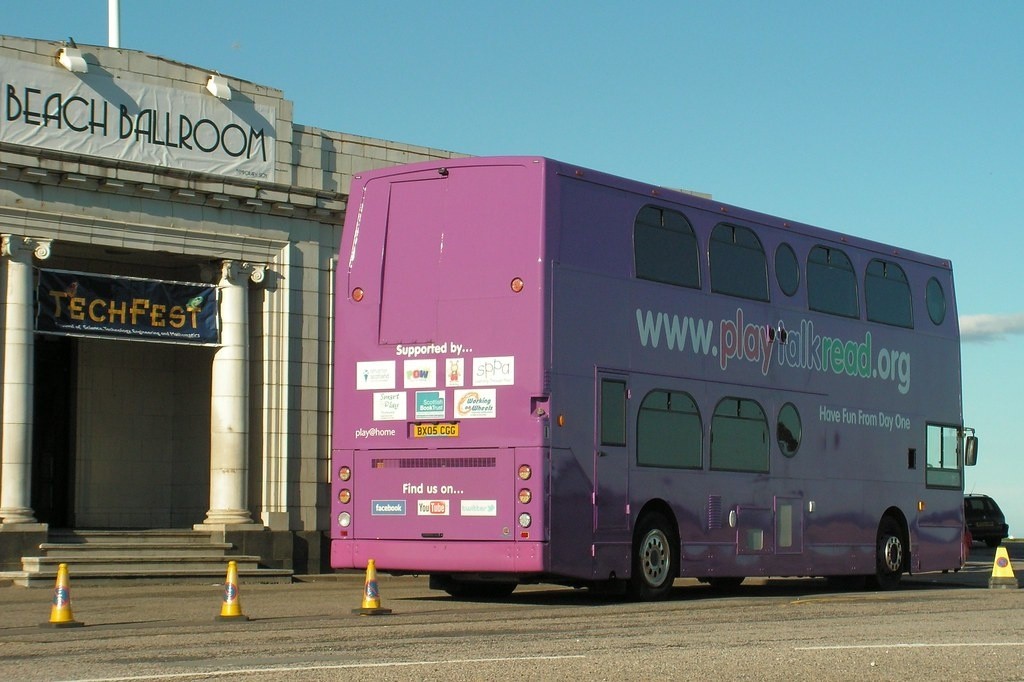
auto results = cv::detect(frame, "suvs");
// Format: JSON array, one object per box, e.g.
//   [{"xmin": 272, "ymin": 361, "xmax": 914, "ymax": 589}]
[{"xmin": 963, "ymin": 494, "xmax": 1009, "ymax": 548}]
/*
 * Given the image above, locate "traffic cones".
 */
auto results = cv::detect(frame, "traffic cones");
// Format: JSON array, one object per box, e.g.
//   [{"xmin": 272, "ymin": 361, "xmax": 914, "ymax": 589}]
[
  {"xmin": 351, "ymin": 559, "xmax": 392, "ymax": 616},
  {"xmin": 49, "ymin": 563, "xmax": 85, "ymax": 627},
  {"xmin": 989, "ymin": 546, "xmax": 1019, "ymax": 589},
  {"xmin": 214, "ymin": 560, "xmax": 249, "ymax": 622}
]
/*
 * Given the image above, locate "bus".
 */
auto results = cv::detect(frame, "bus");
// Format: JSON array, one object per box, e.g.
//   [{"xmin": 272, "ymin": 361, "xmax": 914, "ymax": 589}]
[{"xmin": 330, "ymin": 156, "xmax": 979, "ymax": 599}]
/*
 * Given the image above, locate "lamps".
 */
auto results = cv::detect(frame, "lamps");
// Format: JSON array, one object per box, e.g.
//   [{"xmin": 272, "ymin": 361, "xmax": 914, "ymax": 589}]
[
  {"xmin": 205, "ymin": 76, "xmax": 232, "ymax": 100},
  {"xmin": 58, "ymin": 48, "xmax": 88, "ymax": 73}
]
